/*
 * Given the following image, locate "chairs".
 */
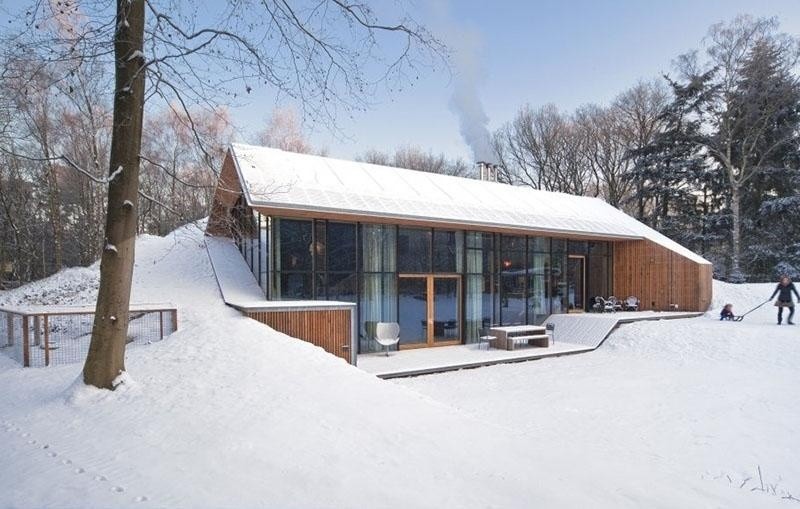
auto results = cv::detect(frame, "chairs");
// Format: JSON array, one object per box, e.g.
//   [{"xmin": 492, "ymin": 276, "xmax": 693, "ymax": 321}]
[
  {"xmin": 444, "ymin": 319, "xmax": 457, "ymax": 338},
  {"xmin": 374, "ymin": 321, "xmax": 402, "ymax": 357},
  {"xmin": 545, "ymin": 321, "xmax": 555, "ymax": 345},
  {"xmin": 421, "ymin": 320, "xmax": 427, "ymax": 339},
  {"xmin": 593, "ymin": 295, "xmax": 641, "ymax": 314},
  {"xmin": 476, "ymin": 326, "xmax": 499, "ymax": 351},
  {"xmin": 359, "ymin": 320, "xmax": 377, "ymax": 352}
]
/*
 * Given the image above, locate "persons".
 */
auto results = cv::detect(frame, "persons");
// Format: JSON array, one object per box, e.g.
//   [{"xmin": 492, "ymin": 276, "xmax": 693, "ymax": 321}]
[
  {"xmin": 769, "ymin": 274, "xmax": 800, "ymax": 326},
  {"xmin": 719, "ymin": 304, "xmax": 734, "ymax": 320},
  {"xmin": 286, "ymin": 253, "xmax": 304, "ymax": 298}
]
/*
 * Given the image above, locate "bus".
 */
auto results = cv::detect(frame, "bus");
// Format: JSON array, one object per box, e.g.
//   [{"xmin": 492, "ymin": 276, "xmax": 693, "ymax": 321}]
[{"xmin": 501, "ymin": 268, "xmax": 562, "ymax": 301}]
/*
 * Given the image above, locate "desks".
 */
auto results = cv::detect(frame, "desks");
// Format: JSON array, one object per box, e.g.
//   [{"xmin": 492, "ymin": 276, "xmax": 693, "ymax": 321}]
[
  {"xmin": 489, "ymin": 324, "xmax": 547, "ymax": 350},
  {"xmin": 434, "ymin": 320, "xmax": 457, "ymax": 337}
]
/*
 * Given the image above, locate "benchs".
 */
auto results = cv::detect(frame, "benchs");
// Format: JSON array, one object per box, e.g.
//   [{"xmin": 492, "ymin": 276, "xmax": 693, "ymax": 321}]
[{"xmin": 507, "ymin": 334, "xmax": 549, "ymax": 350}]
